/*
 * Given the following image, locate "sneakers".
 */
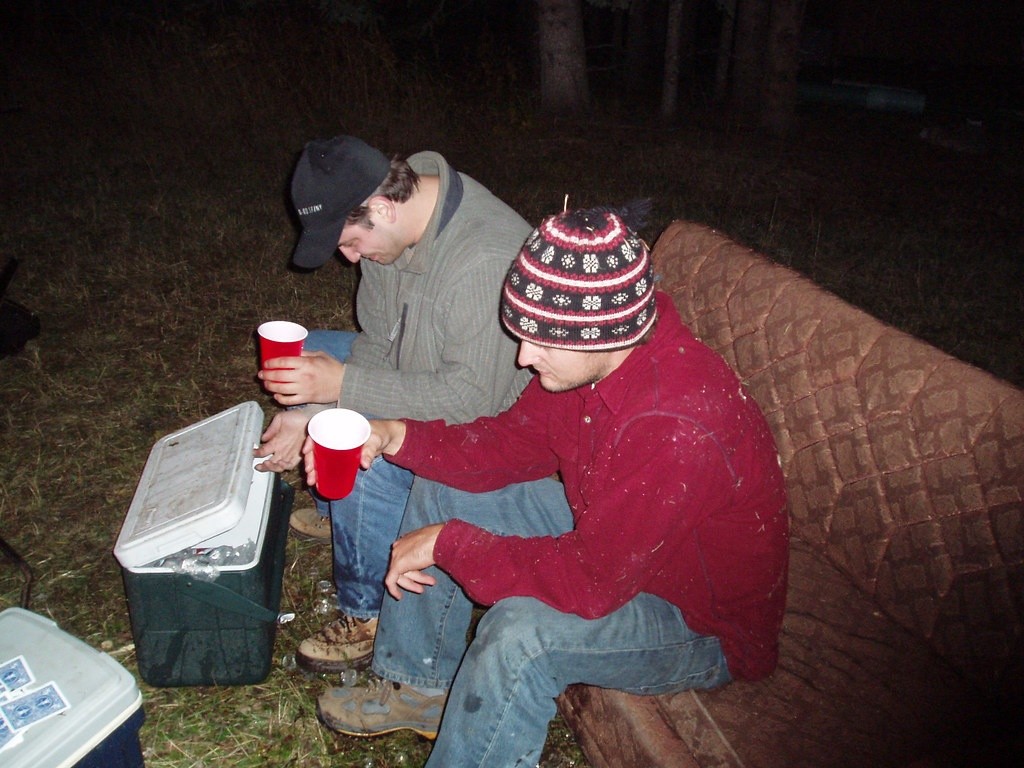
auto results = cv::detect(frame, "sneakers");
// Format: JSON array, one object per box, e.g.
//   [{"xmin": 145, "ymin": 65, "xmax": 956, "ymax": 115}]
[
  {"xmin": 288, "ymin": 508, "xmax": 332, "ymax": 544},
  {"xmin": 316, "ymin": 675, "xmax": 449, "ymax": 745},
  {"xmin": 296, "ymin": 613, "xmax": 378, "ymax": 674}
]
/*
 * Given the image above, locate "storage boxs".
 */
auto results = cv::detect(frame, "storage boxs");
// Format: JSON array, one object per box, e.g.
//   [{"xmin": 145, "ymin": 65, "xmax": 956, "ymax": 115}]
[
  {"xmin": 0, "ymin": 606, "xmax": 147, "ymax": 768},
  {"xmin": 114, "ymin": 401, "xmax": 294, "ymax": 685}
]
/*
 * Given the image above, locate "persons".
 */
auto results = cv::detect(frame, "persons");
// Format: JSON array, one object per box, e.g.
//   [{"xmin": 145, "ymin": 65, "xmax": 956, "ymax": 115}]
[
  {"xmin": 300, "ymin": 204, "xmax": 788, "ymax": 768},
  {"xmin": 252, "ymin": 132, "xmax": 540, "ymax": 671}
]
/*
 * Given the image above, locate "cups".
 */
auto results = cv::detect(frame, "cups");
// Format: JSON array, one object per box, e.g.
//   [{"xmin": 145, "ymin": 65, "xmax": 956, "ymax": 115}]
[
  {"xmin": 307, "ymin": 407, "xmax": 372, "ymax": 499},
  {"xmin": 258, "ymin": 320, "xmax": 309, "ymax": 384}
]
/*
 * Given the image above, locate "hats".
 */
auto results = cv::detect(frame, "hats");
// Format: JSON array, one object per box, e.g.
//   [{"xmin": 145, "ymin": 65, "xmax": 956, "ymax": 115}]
[
  {"xmin": 501, "ymin": 209, "xmax": 657, "ymax": 351},
  {"xmin": 290, "ymin": 136, "xmax": 391, "ymax": 269}
]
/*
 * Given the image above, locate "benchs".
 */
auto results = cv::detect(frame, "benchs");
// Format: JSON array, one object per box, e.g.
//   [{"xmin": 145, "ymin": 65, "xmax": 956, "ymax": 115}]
[{"xmin": 555, "ymin": 218, "xmax": 1024, "ymax": 768}]
